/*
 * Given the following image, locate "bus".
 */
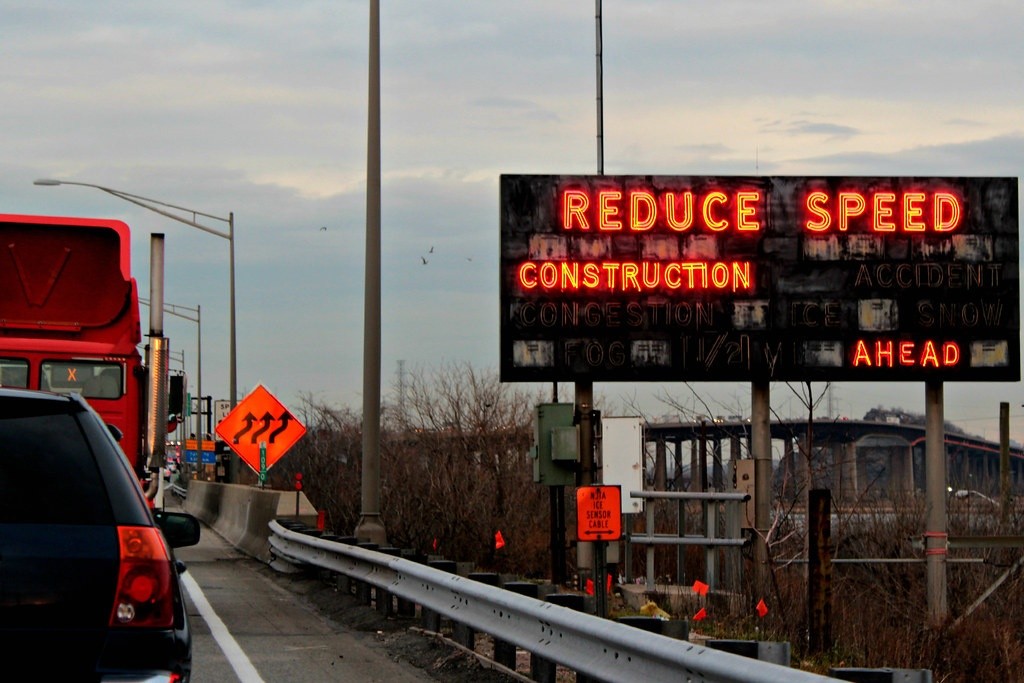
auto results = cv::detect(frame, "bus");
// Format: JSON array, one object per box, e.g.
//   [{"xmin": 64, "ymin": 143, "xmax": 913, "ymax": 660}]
[{"xmin": 0, "ymin": 211, "xmax": 188, "ymax": 512}]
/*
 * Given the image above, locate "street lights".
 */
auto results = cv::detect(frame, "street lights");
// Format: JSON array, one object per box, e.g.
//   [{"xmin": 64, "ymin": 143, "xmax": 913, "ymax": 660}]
[{"xmin": 32, "ymin": 178, "xmax": 238, "ymax": 483}]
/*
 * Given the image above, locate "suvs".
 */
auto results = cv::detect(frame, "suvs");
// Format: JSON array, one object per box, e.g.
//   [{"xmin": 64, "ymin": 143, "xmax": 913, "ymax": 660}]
[{"xmin": 0, "ymin": 385, "xmax": 205, "ymax": 683}]
